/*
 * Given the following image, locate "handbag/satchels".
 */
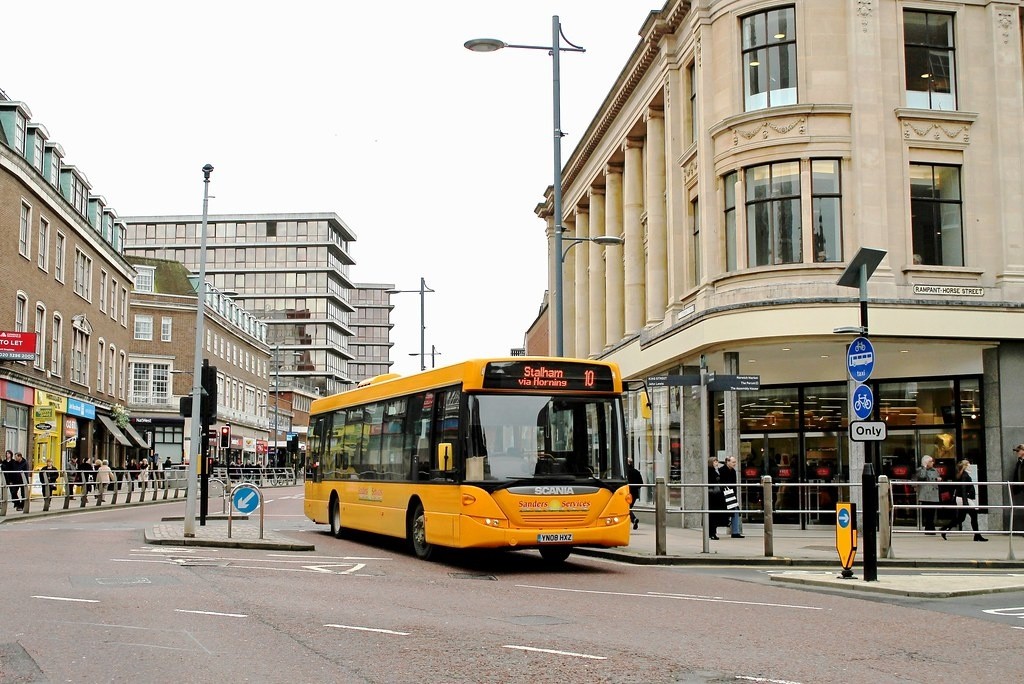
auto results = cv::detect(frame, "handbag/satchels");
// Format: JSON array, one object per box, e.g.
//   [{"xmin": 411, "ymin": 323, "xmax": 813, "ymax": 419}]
[
  {"xmin": 51, "ymin": 483, "xmax": 57, "ymax": 490},
  {"xmin": 723, "ymin": 486, "xmax": 739, "ymax": 510},
  {"xmin": 74, "ymin": 470, "xmax": 82, "ymax": 486}
]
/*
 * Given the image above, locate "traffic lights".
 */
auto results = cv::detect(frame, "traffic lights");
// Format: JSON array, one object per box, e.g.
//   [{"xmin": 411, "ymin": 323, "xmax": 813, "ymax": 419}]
[
  {"xmin": 205, "ymin": 457, "xmax": 213, "ymax": 475},
  {"xmin": 219, "ymin": 426, "xmax": 230, "ymax": 449}
]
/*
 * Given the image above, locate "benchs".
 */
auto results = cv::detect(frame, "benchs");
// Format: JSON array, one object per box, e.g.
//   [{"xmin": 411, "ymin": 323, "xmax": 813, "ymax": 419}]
[{"xmin": 318, "ymin": 470, "xmax": 430, "ymax": 482}]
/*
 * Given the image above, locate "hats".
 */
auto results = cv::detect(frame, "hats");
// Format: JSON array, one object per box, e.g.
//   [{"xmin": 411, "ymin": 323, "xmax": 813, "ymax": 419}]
[{"xmin": 1012, "ymin": 444, "xmax": 1024, "ymax": 451}]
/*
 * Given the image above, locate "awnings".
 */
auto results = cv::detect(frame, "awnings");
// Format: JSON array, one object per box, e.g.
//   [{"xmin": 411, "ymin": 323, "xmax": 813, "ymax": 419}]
[
  {"xmin": 124, "ymin": 423, "xmax": 151, "ymax": 449},
  {"xmin": 97, "ymin": 414, "xmax": 132, "ymax": 447}
]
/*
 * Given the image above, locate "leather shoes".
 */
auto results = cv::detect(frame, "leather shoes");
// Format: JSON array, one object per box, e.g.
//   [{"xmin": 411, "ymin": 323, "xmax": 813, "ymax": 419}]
[
  {"xmin": 731, "ymin": 534, "xmax": 745, "ymax": 538},
  {"xmin": 709, "ymin": 535, "xmax": 719, "ymax": 540}
]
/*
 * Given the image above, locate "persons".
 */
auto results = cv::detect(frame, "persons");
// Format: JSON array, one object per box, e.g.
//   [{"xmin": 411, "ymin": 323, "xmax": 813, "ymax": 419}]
[
  {"xmin": 76, "ymin": 458, "xmax": 94, "ymax": 503},
  {"xmin": 229, "ymin": 458, "xmax": 261, "ymax": 479},
  {"xmin": 720, "ymin": 455, "xmax": 745, "ymax": 538},
  {"xmin": 211, "ymin": 458, "xmax": 226, "ymax": 474},
  {"xmin": 916, "ymin": 455, "xmax": 942, "ymax": 535},
  {"xmin": 93, "ymin": 459, "xmax": 102, "ymax": 499},
  {"xmin": 39, "ymin": 459, "xmax": 59, "ymax": 507},
  {"xmin": 1012, "ymin": 444, "xmax": 1024, "ymax": 505},
  {"xmin": 164, "ymin": 456, "xmax": 171, "ymax": 467},
  {"xmin": 627, "ymin": 457, "xmax": 643, "ymax": 530},
  {"xmin": 127, "ymin": 458, "xmax": 149, "ymax": 480},
  {"xmin": 913, "ymin": 254, "xmax": 922, "ymax": 265},
  {"xmin": 818, "ymin": 250, "xmax": 829, "ymax": 261},
  {"xmin": 67, "ymin": 456, "xmax": 78, "ymax": 500},
  {"xmin": 708, "ymin": 457, "xmax": 720, "ymax": 540},
  {"xmin": 940, "ymin": 460, "xmax": 989, "ymax": 542},
  {"xmin": 1, "ymin": 449, "xmax": 29, "ymax": 511},
  {"xmin": 95, "ymin": 460, "xmax": 113, "ymax": 502}
]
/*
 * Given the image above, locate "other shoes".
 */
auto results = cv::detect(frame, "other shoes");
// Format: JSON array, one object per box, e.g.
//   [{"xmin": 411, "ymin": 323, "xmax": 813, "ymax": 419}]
[
  {"xmin": 16, "ymin": 507, "xmax": 23, "ymax": 511},
  {"xmin": 84, "ymin": 498, "xmax": 89, "ymax": 503},
  {"xmin": 101, "ymin": 500, "xmax": 106, "ymax": 502},
  {"xmin": 70, "ymin": 497, "xmax": 76, "ymax": 500}
]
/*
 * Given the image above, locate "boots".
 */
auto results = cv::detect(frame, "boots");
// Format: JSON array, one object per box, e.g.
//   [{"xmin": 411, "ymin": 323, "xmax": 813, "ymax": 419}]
[
  {"xmin": 939, "ymin": 524, "xmax": 952, "ymax": 540},
  {"xmin": 974, "ymin": 533, "xmax": 988, "ymax": 541}
]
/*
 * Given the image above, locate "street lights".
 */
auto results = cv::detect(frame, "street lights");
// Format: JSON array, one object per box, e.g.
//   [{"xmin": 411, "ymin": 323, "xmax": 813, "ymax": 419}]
[
  {"xmin": 463, "ymin": 15, "xmax": 625, "ymax": 450},
  {"xmin": 258, "ymin": 346, "xmax": 303, "ymax": 468},
  {"xmin": 176, "ymin": 162, "xmax": 240, "ymax": 537},
  {"xmin": 384, "ymin": 277, "xmax": 436, "ymax": 371},
  {"xmin": 408, "ymin": 344, "xmax": 441, "ymax": 368},
  {"xmin": 830, "ymin": 248, "xmax": 890, "ymax": 581}
]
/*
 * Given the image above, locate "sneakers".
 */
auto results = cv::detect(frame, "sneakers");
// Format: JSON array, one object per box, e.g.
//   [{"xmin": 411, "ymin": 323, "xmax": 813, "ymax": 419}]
[{"xmin": 633, "ymin": 519, "xmax": 639, "ymax": 530}]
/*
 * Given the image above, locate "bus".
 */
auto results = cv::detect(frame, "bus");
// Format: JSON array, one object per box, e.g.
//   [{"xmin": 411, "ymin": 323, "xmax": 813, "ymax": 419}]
[{"xmin": 303, "ymin": 357, "xmax": 653, "ymax": 564}]
